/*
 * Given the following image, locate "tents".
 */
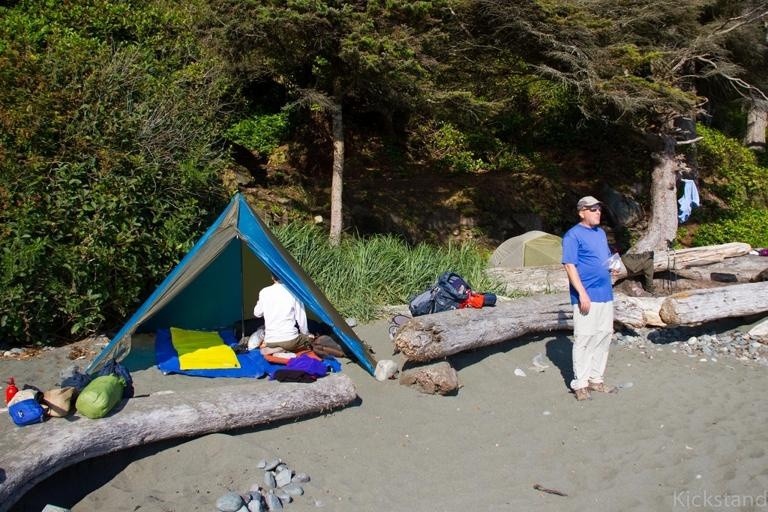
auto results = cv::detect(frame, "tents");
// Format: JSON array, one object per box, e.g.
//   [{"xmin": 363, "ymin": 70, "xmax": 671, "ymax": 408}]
[{"xmin": 85, "ymin": 193, "xmax": 379, "ymax": 376}]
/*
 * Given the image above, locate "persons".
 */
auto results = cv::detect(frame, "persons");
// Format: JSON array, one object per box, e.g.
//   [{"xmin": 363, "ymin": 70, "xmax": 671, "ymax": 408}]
[
  {"xmin": 253, "ymin": 273, "xmax": 315, "ymax": 357},
  {"xmin": 562, "ymin": 196, "xmax": 628, "ymax": 400}
]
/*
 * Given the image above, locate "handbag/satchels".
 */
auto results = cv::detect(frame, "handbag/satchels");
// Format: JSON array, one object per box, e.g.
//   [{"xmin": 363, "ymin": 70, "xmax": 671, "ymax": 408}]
[
  {"xmin": 8, "ymin": 384, "xmax": 50, "ymax": 427},
  {"xmin": 61, "ymin": 358, "xmax": 135, "ymax": 398},
  {"xmin": 42, "ymin": 387, "xmax": 74, "ymax": 418}
]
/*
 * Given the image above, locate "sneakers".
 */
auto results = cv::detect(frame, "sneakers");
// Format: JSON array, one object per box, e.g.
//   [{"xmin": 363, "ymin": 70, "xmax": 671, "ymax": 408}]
[
  {"xmin": 588, "ymin": 383, "xmax": 616, "ymax": 392},
  {"xmin": 575, "ymin": 387, "xmax": 592, "ymax": 401}
]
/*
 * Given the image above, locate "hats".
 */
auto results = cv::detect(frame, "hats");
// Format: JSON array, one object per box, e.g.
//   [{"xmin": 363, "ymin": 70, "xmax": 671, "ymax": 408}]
[{"xmin": 577, "ymin": 195, "xmax": 604, "ymax": 211}]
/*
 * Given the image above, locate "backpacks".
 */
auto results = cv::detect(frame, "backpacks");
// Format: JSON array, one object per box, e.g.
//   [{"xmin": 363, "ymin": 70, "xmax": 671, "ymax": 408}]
[{"xmin": 409, "ymin": 271, "xmax": 471, "ymax": 318}]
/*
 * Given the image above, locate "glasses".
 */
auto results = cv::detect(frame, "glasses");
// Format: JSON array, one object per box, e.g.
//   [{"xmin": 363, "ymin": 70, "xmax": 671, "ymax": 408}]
[{"xmin": 582, "ymin": 207, "xmax": 602, "ymax": 212}]
